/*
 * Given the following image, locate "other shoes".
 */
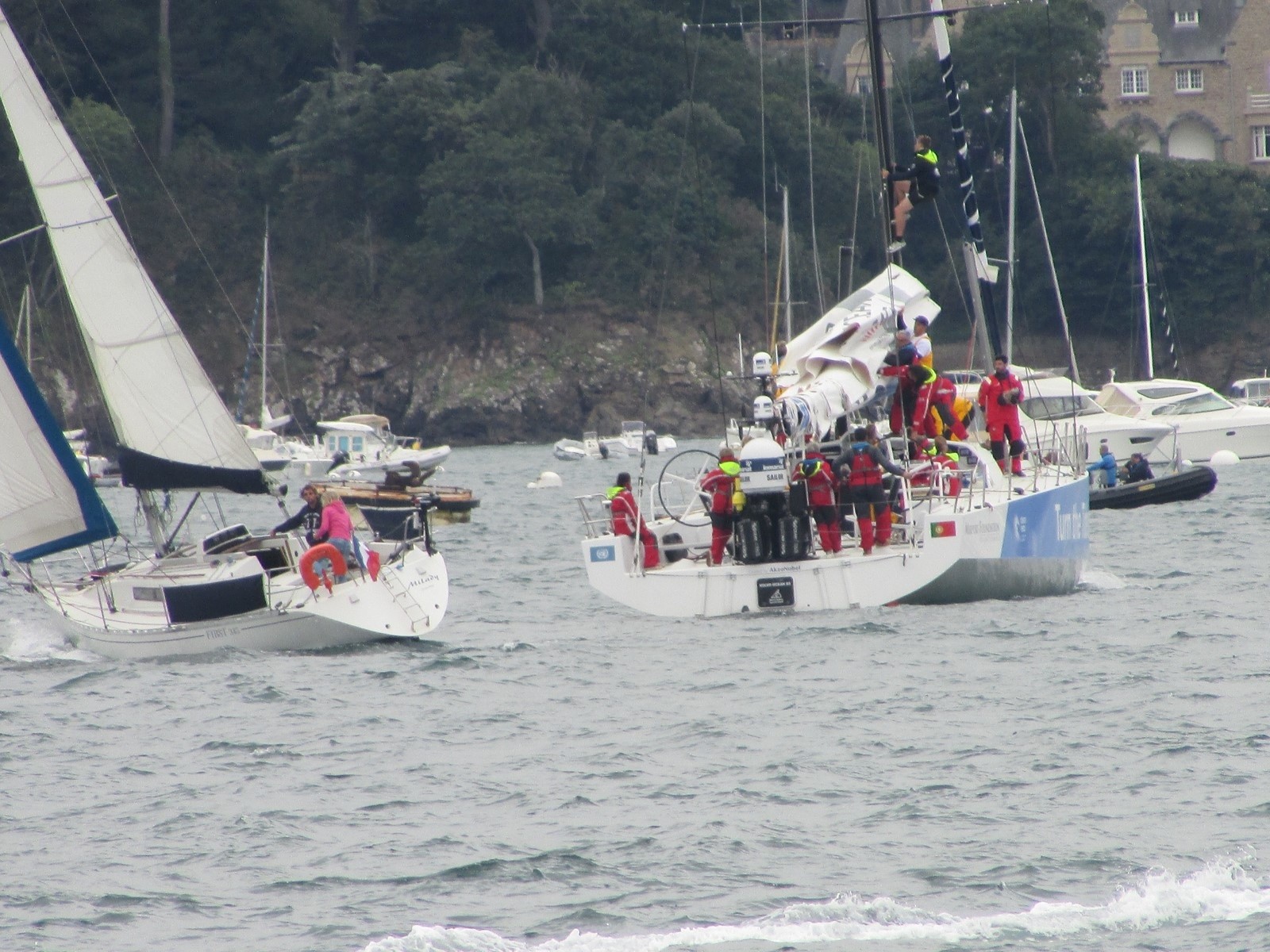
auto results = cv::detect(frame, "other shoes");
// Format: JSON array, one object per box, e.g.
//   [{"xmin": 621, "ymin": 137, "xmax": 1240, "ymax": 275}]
[
  {"xmin": 1003, "ymin": 471, "xmax": 1010, "ymax": 476},
  {"xmin": 863, "ymin": 552, "xmax": 871, "ymax": 556},
  {"xmin": 876, "ymin": 539, "xmax": 891, "ymax": 548},
  {"xmin": 645, "ymin": 563, "xmax": 665, "ymax": 571},
  {"xmin": 1013, "ymin": 470, "xmax": 1027, "ymax": 477},
  {"xmin": 824, "ymin": 553, "xmax": 832, "ymax": 559},
  {"xmin": 712, "ymin": 564, "xmax": 721, "ymax": 567},
  {"xmin": 834, "ymin": 551, "xmax": 850, "ymax": 557}
]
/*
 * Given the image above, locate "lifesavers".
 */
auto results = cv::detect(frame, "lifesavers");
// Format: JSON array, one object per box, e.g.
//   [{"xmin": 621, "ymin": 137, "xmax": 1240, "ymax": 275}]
[{"xmin": 299, "ymin": 542, "xmax": 348, "ymax": 589}]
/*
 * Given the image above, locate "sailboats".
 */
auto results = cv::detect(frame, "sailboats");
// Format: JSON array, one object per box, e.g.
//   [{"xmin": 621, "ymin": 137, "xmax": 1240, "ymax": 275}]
[
  {"xmin": 10, "ymin": 281, "xmax": 109, "ymax": 489},
  {"xmin": 0, "ymin": 0, "xmax": 452, "ymax": 659},
  {"xmin": 573, "ymin": 1, "xmax": 1090, "ymax": 619},
  {"xmin": 770, "ymin": 84, "xmax": 1270, "ymax": 511}
]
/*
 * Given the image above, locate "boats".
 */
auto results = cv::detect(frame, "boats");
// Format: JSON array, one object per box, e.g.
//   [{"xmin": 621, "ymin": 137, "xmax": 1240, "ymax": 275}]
[
  {"xmin": 552, "ymin": 430, "xmax": 630, "ymax": 462},
  {"xmin": 230, "ymin": 203, "xmax": 483, "ymax": 523},
  {"xmin": 596, "ymin": 420, "xmax": 678, "ymax": 457}
]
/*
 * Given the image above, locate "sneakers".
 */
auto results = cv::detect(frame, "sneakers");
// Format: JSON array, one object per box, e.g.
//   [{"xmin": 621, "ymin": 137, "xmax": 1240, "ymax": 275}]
[
  {"xmin": 886, "ymin": 238, "xmax": 906, "ymax": 253},
  {"xmin": 890, "ymin": 214, "xmax": 910, "ymax": 224}
]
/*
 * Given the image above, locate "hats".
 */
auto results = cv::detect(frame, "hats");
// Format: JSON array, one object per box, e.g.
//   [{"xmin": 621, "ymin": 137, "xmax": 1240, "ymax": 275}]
[
  {"xmin": 807, "ymin": 444, "xmax": 820, "ymax": 453},
  {"xmin": 913, "ymin": 315, "xmax": 928, "ymax": 325},
  {"xmin": 719, "ymin": 448, "xmax": 734, "ymax": 457}
]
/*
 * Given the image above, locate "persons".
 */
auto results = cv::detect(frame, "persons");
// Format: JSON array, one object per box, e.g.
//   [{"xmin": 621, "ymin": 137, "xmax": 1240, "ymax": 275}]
[
  {"xmin": 742, "ymin": 435, "xmax": 755, "ymax": 448},
  {"xmin": 701, "ymin": 449, "xmax": 741, "ymax": 566},
  {"xmin": 877, "ymin": 307, "xmax": 969, "ymax": 489},
  {"xmin": 269, "ymin": 485, "xmax": 328, "ymax": 547},
  {"xmin": 882, "ymin": 135, "xmax": 940, "ymax": 253},
  {"xmin": 607, "ymin": 472, "xmax": 663, "ymax": 570},
  {"xmin": 1123, "ymin": 453, "xmax": 1154, "ymax": 484},
  {"xmin": 791, "ymin": 442, "xmax": 842, "ymax": 556},
  {"xmin": 1086, "ymin": 445, "xmax": 1117, "ymax": 488},
  {"xmin": 1043, "ymin": 452, "xmax": 1058, "ymax": 465},
  {"xmin": 313, "ymin": 490, "xmax": 353, "ymax": 585},
  {"xmin": 978, "ymin": 355, "xmax": 1026, "ymax": 478},
  {"xmin": 832, "ymin": 427, "xmax": 912, "ymax": 556}
]
